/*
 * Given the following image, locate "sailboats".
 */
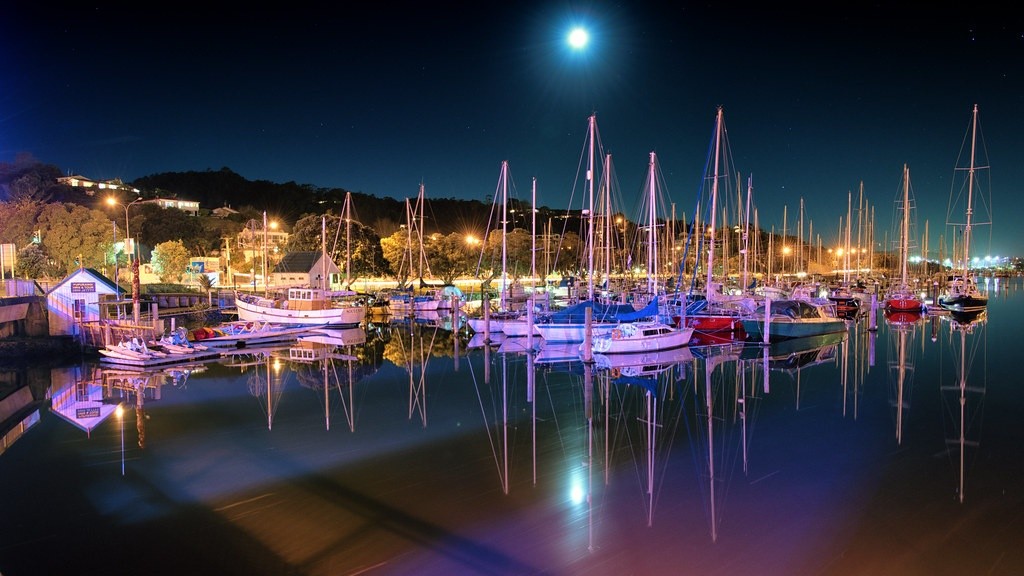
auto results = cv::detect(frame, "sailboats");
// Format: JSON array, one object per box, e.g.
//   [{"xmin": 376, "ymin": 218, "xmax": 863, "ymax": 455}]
[{"xmin": 97, "ymin": 103, "xmax": 989, "ymax": 370}]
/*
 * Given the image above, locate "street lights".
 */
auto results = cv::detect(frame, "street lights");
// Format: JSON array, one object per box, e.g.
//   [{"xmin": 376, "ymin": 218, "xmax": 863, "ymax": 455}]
[
  {"xmin": 260, "ymin": 221, "xmax": 279, "ymax": 286},
  {"xmin": 106, "ymin": 196, "xmax": 143, "ymax": 267}
]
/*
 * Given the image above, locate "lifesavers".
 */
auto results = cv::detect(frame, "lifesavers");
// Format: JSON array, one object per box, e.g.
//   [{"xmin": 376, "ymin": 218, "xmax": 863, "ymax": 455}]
[
  {"xmin": 611, "ymin": 329, "xmax": 622, "ymax": 339},
  {"xmin": 611, "ymin": 368, "xmax": 621, "ymax": 378}
]
